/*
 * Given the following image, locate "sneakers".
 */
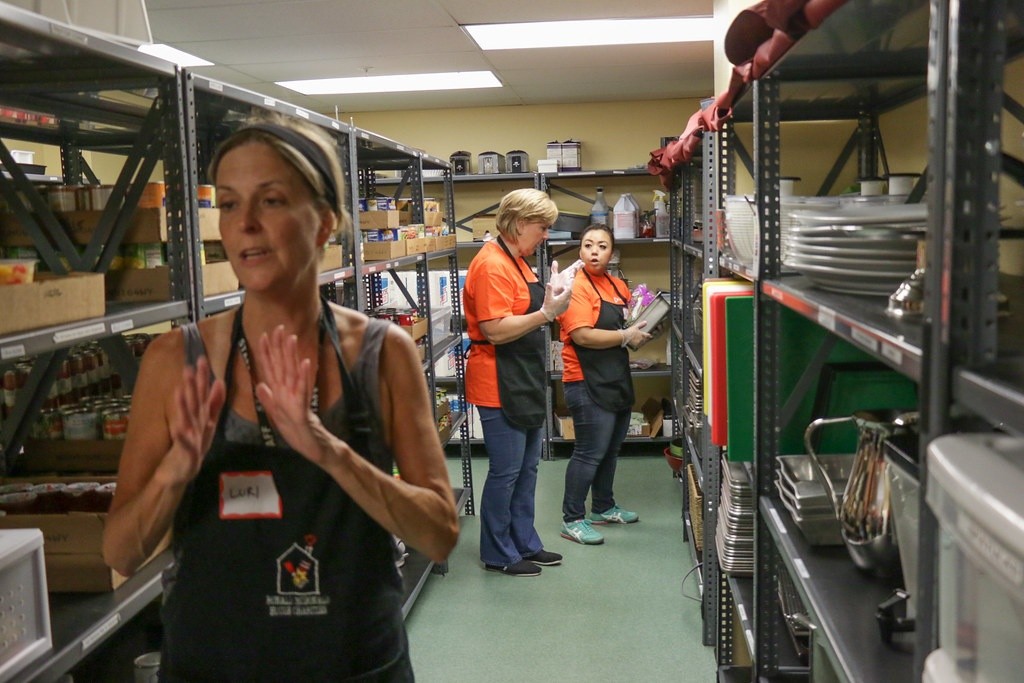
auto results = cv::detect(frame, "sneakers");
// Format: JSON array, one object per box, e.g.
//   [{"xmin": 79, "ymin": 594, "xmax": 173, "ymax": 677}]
[
  {"xmin": 590, "ymin": 504, "xmax": 639, "ymax": 524},
  {"xmin": 560, "ymin": 515, "xmax": 604, "ymax": 545}
]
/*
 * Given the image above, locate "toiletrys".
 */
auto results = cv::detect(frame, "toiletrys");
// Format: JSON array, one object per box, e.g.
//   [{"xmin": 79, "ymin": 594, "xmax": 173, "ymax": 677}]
[{"xmin": 651, "ymin": 188, "xmax": 670, "ymax": 238}]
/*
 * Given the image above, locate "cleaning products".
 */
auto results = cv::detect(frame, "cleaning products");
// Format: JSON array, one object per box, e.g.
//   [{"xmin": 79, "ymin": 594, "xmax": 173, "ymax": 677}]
[
  {"xmin": 612, "ymin": 193, "xmax": 640, "ymax": 239},
  {"xmin": 591, "ymin": 186, "xmax": 608, "ymax": 228}
]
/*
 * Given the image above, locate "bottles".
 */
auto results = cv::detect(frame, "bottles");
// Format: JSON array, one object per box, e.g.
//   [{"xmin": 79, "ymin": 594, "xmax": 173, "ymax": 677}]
[
  {"xmin": 612, "ymin": 193, "xmax": 638, "ymax": 240},
  {"xmin": 591, "ymin": 187, "xmax": 609, "ymax": 227}
]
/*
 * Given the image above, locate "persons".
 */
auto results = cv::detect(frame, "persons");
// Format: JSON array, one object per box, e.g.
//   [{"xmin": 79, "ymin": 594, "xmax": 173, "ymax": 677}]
[
  {"xmin": 102, "ymin": 121, "xmax": 460, "ymax": 683},
  {"xmin": 557, "ymin": 223, "xmax": 652, "ymax": 545},
  {"xmin": 462, "ymin": 188, "xmax": 586, "ymax": 576}
]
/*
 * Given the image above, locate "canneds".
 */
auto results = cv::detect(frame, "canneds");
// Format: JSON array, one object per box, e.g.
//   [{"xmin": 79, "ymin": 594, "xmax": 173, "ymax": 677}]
[
  {"xmin": 0, "ymin": 332, "xmax": 167, "ymax": 442},
  {"xmin": 0, "ymin": 243, "xmax": 225, "ymax": 274},
  {"xmin": 361, "ymin": 307, "xmax": 419, "ymax": 327},
  {"xmin": 33, "ymin": 179, "xmax": 217, "ymax": 209},
  {"xmin": 133, "ymin": 651, "xmax": 161, "ymax": 683},
  {"xmin": 436, "ymin": 388, "xmax": 452, "ymax": 432}
]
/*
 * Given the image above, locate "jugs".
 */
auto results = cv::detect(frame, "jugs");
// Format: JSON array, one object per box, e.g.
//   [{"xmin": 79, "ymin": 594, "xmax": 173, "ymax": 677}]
[{"xmin": 804, "ymin": 408, "xmax": 921, "ymax": 577}]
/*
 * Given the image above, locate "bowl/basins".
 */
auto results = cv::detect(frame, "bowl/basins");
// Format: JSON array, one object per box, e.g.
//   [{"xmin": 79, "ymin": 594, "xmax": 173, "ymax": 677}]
[{"xmin": 719, "ymin": 194, "xmax": 837, "ymax": 275}]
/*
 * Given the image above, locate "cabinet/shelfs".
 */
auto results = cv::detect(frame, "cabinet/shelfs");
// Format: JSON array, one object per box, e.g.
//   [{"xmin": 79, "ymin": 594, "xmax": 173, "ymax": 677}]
[
  {"xmin": 0, "ymin": 1, "xmax": 474, "ymax": 683},
  {"xmin": 361, "ymin": 166, "xmax": 670, "ymax": 460},
  {"xmin": 682, "ymin": 0, "xmax": 1024, "ymax": 683}
]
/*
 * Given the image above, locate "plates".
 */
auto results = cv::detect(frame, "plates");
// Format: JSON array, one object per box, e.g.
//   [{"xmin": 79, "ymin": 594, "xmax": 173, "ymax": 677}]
[{"xmin": 785, "ymin": 196, "xmax": 931, "ymax": 299}]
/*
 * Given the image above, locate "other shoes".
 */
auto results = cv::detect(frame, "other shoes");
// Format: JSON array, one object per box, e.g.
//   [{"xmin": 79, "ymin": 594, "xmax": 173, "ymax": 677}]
[
  {"xmin": 485, "ymin": 560, "xmax": 543, "ymax": 577},
  {"xmin": 522, "ymin": 549, "xmax": 562, "ymax": 565}
]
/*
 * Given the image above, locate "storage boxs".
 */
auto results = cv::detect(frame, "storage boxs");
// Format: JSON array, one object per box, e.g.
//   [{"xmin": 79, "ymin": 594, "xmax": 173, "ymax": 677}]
[
  {"xmin": 397, "ymin": 318, "xmax": 429, "ymax": 340},
  {"xmin": 553, "ymin": 408, "xmax": 576, "ymax": 440},
  {"xmin": 436, "ymin": 235, "xmax": 458, "ymax": 251},
  {"xmin": 360, "ymin": 210, "xmax": 399, "ymax": 230},
  {"xmin": 319, "ymin": 244, "xmax": 343, "ymax": 273},
  {"xmin": 406, "ymin": 237, "xmax": 437, "ymax": 254},
  {"xmin": 400, "ymin": 202, "xmax": 443, "ymax": 226},
  {"xmin": 627, "ymin": 424, "xmax": 650, "ymax": 437},
  {"xmin": 107, "ymin": 260, "xmax": 239, "ymax": 299},
  {"xmin": 922, "ymin": 433, "xmax": 1024, "ymax": 683},
  {"xmin": 0, "ymin": 513, "xmax": 172, "ymax": 595},
  {"xmin": 363, "ymin": 240, "xmax": 406, "ymax": 260},
  {"xmin": 0, "ymin": 208, "xmax": 222, "ymax": 245},
  {"xmin": 22, "ymin": 438, "xmax": 123, "ymax": 474},
  {"xmin": 0, "ymin": 272, "xmax": 105, "ymax": 332}
]
